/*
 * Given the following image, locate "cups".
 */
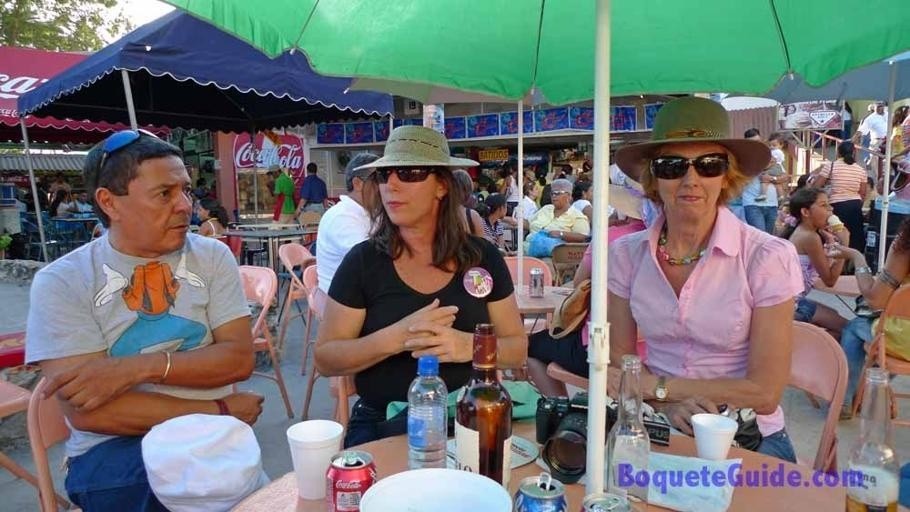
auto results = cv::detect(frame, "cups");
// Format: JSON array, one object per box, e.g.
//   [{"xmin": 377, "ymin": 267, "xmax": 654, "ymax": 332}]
[
  {"xmin": 286, "ymin": 417, "xmax": 344, "ymax": 500},
  {"xmin": 692, "ymin": 412, "xmax": 738, "ymax": 459}
]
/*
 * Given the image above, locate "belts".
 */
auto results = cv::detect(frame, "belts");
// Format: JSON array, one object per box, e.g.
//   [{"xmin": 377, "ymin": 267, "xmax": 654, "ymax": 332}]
[{"xmin": 305, "ymin": 199, "xmax": 324, "ymax": 204}]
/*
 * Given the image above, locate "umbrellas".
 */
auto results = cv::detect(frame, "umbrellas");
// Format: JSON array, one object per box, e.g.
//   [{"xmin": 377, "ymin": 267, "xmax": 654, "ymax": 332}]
[
  {"xmin": 766, "ymin": 48, "xmax": 910, "ymax": 276},
  {"xmin": 159, "ymin": 0, "xmax": 910, "ymax": 512}
]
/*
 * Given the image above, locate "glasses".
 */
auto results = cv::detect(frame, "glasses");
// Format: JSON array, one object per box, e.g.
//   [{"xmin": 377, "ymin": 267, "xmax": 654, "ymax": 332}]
[
  {"xmin": 648, "ymin": 152, "xmax": 734, "ymax": 185},
  {"xmin": 890, "ymin": 168, "xmax": 910, "ymax": 192},
  {"xmin": 91, "ymin": 124, "xmax": 164, "ymax": 189},
  {"xmin": 371, "ymin": 159, "xmax": 440, "ymax": 186},
  {"xmin": 550, "ymin": 189, "xmax": 570, "ymax": 198}
]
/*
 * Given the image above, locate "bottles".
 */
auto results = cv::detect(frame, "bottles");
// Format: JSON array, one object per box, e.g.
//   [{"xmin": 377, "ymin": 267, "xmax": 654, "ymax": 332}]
[
  {"xmin": 605, "ymin": 353, "xmax": 652, "ymax": 511},
  {"xmin": 845, "ymin": 367, "xmax": 901, "ymax": 511},
  {"xmin": 455, "ymin": 323, "xmax": 514, "ymax": 488},
  {"xmin": 407, "ymin": 353, "xmax": 447, "ymax": 472}
]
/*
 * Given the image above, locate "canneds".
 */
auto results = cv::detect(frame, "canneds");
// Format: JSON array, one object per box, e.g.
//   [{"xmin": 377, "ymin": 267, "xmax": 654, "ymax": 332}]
[
  {"xmin": 719, "ymin": 404, "xmax": 739, "ymax": 447},
  {"xmin": 529, "ymin": 266, "xmax": 544, "ymax": 298},
  {"xmin": 512, "ymin": 472, "xmax": 569, "ymax": 512},
  {"xmin": 326, "ymin": 451, "xmax": 377, "ymax": 512},
  {"xmin": 579, "ymin": 493, "xmax": 630, "ymax": 512}
]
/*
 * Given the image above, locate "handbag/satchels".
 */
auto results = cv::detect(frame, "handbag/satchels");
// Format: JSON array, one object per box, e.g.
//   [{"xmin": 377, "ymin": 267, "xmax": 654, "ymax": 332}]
[
  {"xmin": 853, "ymin": 291, "xmax": 885, "ymax": 320},
  {"xmin": 524, "ymin": 227, "xmax": 571, "ymax": 260},
  {"xmin": 821, "ymin": 159, "xmax": 837, "ymax": 199},
  {"xmin": 547, "ymin": 276, "xmax": 592, "ymax": 340}
]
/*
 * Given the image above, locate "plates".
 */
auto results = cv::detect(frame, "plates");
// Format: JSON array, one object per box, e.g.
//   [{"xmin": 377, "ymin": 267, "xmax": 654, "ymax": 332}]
[{"xmin": 359, "ymin": 468, "xmax": 513, "ymax": 512}]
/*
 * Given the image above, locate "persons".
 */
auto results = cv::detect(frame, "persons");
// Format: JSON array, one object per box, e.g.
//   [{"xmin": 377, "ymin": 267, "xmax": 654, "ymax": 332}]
[{"xmin": 23, "ymin": 97, "xmax": 910, "ymax": 512}]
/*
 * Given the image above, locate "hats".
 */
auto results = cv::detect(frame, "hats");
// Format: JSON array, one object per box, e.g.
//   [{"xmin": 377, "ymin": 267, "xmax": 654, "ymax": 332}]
[
  {"xmin": 350, "ymin": 123, "xmax": 483, "ymax": 174},
  {"xmin": 873, "ymin": 159, "xmax": 910, "ymax": 215},
  {"xmin": 134, "ymin": 409, "xmax": 275, "ymax": 512},
  {"xmin": 612, "ymin": 94, "xmax": 777, "ymax": 191},
  {"xmin": 607, "ymin": 175, "xmax": 647, "ymax": 222},
  {"xmin": 266, "ymin": 164, "xmax": 282, "ymax": 176}
]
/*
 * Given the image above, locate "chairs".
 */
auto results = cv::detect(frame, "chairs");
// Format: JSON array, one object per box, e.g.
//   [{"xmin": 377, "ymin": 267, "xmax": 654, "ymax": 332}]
[{"xmin": 0, "ymin": 209, "xmax": 910, "ymax": 511}]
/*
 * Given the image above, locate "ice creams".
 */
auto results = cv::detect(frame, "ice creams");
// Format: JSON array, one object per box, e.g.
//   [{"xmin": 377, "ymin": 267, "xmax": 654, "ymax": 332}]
[{"xmin": 827, "ymin": 215, "xmax": 843, "ymax": 233}]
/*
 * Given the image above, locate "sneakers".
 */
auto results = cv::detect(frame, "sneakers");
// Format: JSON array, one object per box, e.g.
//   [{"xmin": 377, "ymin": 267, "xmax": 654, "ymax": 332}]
[
  {"xmin": 777, "ymin": 195, "xmax": 786, "ymax": 203},
  {"xmin": 851, "ymin": 386, "xmax": 899, "ymax": 420},
  {"xmin": 754, "ymin": 193, "xmax": 769, "ymax": 202}
]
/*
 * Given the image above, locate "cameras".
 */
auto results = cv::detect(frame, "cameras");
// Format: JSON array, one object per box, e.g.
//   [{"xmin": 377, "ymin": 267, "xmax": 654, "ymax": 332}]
[{"xmin": 535, "ymin": 394, "xmax": 615, "ymax": 485}]
